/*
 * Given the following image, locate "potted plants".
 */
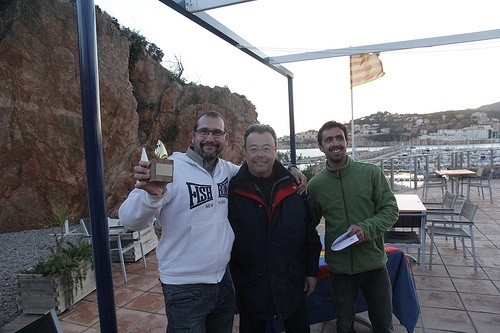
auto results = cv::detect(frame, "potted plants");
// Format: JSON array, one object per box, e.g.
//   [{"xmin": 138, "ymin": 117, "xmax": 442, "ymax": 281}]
[
  {"xmin": 110, "ymin": 225, "xmax": 159, "ymax": 261},
  {"xmin": 16, "ymin": 203, "xmax": 96, "ymax": 314}
]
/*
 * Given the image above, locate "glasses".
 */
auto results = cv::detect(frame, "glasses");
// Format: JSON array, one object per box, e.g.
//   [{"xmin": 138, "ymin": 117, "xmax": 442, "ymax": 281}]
[
  {"xmin": 193, "ymin": 128, "xmax": 226, "ymax": 136},
  {"xmin": 244, "ymin": 146, "xmax": 277, "ymax": 154}
]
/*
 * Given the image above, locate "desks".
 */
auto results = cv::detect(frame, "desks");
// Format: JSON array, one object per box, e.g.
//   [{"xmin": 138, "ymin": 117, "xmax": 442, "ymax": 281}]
[
  {"xmin": 308, "ymin": 233, "xmax": 420, "ymax": 333},
  {"xmin": 433, "ymin": 169, "xmax": 476, "ymax": 202},
  {"xmin": 383, "ymin": 194, "xmax": 428, "ymax": 280}
]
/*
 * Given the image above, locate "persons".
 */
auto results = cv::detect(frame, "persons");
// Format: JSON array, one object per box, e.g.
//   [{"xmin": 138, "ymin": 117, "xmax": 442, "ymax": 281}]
[
  {"xmin": 118, "ymin": 111, "xmax": 307, "ymax": 333},
  {"xmin": 305, "ymin": 121, "xmax": 399, "ymax": 333},
  {"xmin": 153, "ymin": 124, "xmax": 323, "ymax": 333}
]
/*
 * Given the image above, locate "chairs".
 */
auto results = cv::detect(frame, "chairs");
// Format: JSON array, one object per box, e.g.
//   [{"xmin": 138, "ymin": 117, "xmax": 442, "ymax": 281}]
[
  {"xmin": 79, "ymin": 218, "xmax": 146, "ymax": 284},
  {"xmin": 384, "ymin": 166, "xmax": 494, "ymax": 270}
]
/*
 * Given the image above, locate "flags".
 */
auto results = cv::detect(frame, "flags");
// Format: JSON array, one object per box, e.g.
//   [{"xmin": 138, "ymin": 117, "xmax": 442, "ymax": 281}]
[{"xmin": 351, "ymin": 52, "xmax": 385, "ymax": 88}]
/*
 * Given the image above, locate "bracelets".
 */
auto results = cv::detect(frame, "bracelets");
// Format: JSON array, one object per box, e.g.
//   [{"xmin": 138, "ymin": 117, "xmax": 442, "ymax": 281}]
[{"xmin": 288, "ymin": 164, "xmax": 297, "ymax": 171}]
